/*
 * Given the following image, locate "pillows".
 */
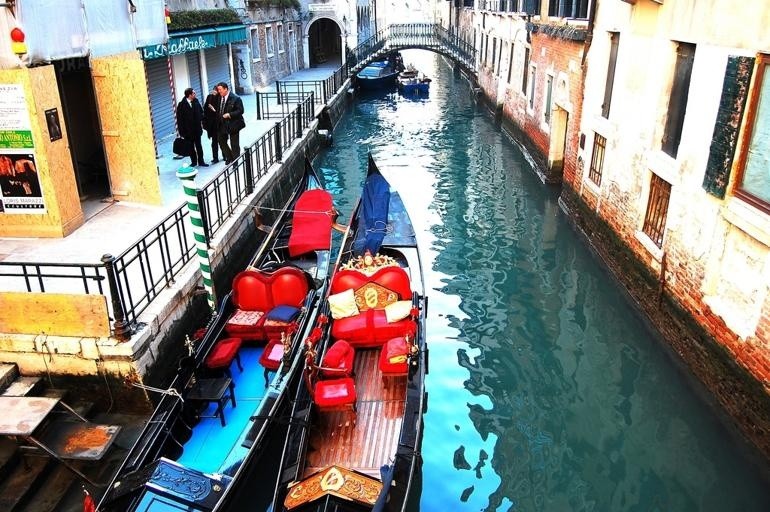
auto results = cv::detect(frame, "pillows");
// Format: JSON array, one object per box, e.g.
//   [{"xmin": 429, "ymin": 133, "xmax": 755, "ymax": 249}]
[
  {"xmin": 328, "ymin": 289, "xmax": 359, "ymax": 319},
  {"xmin": 266, "ymin": 304, "xmax": 301, "ymax": 324},
  {"xmin": 384, "ymin": 299, "xmax": 413, "ymax": 324}
]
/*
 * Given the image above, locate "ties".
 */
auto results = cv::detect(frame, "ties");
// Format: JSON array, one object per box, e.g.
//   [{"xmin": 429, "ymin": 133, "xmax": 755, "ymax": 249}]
[{"xmin": 221, "ymin": 96, "xmax": 225, "ymax": 114}]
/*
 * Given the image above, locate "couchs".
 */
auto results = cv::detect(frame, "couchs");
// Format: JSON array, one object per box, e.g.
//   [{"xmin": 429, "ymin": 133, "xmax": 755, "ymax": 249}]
[
  {"xmin": 225, "ymin": 265, "xmax": 310, "ymax": 341},
  {"xmin": 328, "ymin": 251, "xmax": 416, "ymax": 345}
]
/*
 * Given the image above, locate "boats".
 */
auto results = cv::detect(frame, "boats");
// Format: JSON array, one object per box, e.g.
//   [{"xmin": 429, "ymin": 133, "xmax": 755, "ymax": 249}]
[
  {"xmin": 356, "ymin": 53, "xmax": 404, "ymax": 89},
  {"xmin": 270, "ymin": 146, "xmax": 426, "ymax": 512},
  {"xmin": 397, "ymin": 63, "xmax": 432, "ymax": 94},
  {"xmin": 94, "ymin": 154, "xmax": 336, "ymax": 512}
]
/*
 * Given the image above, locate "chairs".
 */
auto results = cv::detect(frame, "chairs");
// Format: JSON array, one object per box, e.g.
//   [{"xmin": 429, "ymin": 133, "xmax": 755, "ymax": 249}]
[
  {"xmin": 178, "ymin": 354, "xmax": 236, "ymax": 429},
  {"xmin": 302, "ymin": 355, "xmax": 360, "ymax": 420}
]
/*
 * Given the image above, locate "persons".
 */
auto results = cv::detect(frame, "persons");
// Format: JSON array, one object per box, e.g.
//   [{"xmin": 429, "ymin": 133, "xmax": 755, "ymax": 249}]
[
  {"xmin": 202, "ymin": 85, "xmax": 227, "ymax": 164},
  {"xmin": 49, "ymin": 114, "xmax": 61, "ymax": 141},
  {"xmin": 175, "ymin": 87, "xmax": 209, "ymax": 168},
  {"xmin": 208, "ymin": 82, "xmax": 246, "ymax": 170}
]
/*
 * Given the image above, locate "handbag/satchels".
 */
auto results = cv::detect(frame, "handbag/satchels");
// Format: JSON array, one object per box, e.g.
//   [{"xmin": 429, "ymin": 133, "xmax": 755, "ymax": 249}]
[{"xmin": 173, "ymin": 138, "xmax": 190, "ymax": 156}]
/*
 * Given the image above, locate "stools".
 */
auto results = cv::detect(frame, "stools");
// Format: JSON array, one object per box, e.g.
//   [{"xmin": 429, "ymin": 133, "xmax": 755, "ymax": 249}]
[
  {"xmin": 261, "ymin": 338, "xmax": 285, "ymax": 388},
  {"xmin": 323, "ymin": 346, "xmax": 355, "ymax": 379},
  {"xmin": 377, "ymin": 339, "xmax": 408, "ymax": 388},
  {"xmin": 208, "ymin": 338, "xmax": 243, "ymax": 375}
]
